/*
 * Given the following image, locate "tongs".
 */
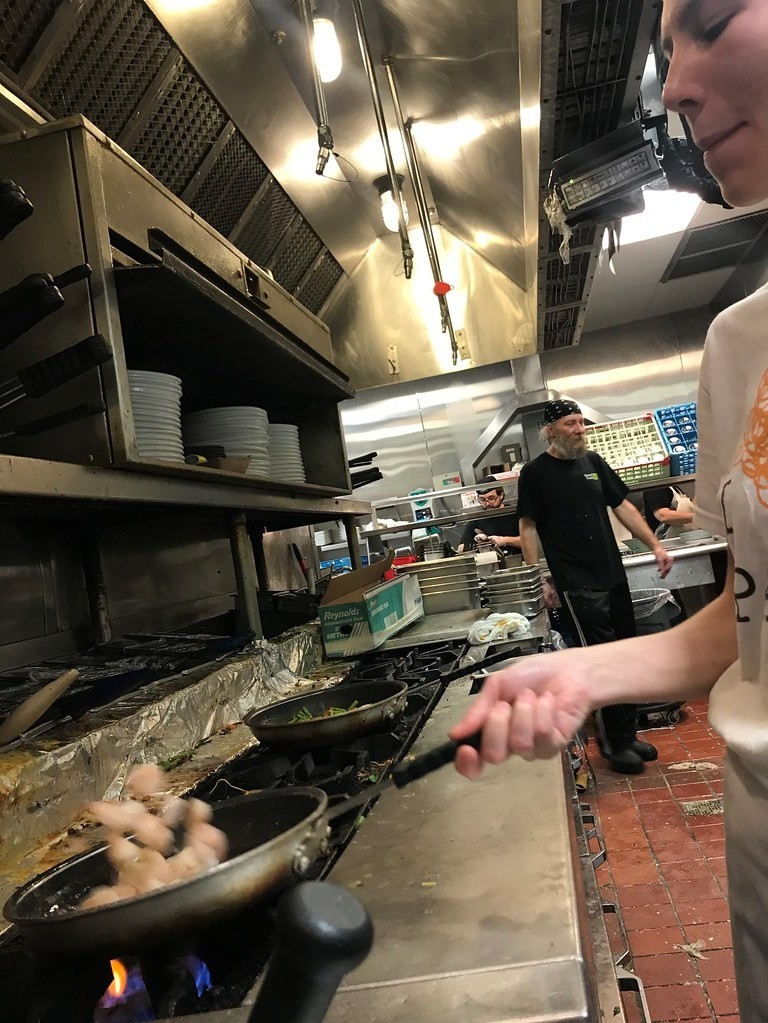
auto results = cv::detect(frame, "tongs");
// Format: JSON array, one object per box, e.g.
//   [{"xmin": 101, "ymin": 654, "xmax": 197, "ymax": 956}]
[{"xmin": 473, "ymin": 527, "xmax": 505, "ymax": 558}]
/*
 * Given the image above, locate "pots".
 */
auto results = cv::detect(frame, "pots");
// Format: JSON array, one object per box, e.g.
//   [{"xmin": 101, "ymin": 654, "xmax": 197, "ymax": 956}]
[
  {"xmin": 1, "ymin": 724, "xmax": 489, "ymax": 952},
  {"xmin": 240, "ymin": 644, "xmax": 523, "ymax": 750}
]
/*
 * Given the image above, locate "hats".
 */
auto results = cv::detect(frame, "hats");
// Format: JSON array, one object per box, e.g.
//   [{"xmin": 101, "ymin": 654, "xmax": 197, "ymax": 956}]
[{"xmin": 475, "ymin": 476, "xmax": 498, "ymax": 494}]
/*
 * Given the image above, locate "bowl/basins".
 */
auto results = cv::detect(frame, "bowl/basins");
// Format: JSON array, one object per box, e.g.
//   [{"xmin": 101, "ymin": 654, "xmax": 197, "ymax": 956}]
[{"xmin": 621, "ymin": 537, "xmax": 654, "ymax": 553}]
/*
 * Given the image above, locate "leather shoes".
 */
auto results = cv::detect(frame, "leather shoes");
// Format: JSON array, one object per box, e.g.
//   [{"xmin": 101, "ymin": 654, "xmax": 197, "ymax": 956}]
[
  {"xmin": 602, "ymin": 750, "xmax": 643, "ymax": 773},
  {"xmin": 632, "ymin": 741, "xmax": 658, "ymax": 760}
]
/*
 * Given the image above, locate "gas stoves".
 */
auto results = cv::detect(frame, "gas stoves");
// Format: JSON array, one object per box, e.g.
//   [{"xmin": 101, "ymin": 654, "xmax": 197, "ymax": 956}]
[{"xmin": 0, "ymin": 636, "xmax": 473, "ymax": 1023}]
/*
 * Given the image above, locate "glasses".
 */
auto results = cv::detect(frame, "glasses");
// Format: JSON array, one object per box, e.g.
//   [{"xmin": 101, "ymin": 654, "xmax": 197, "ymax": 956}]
[{"xmin": 478, "ymin": 495, "xmax": 499, "ymax": 504}]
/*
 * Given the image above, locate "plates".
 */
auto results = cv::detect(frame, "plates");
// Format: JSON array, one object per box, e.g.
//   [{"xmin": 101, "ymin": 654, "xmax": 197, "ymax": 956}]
[
  {"xmin": 312, "ymin": 522, "xmax": 364, "ymax": 548},
  {"xmin": 656, "ymin": 530, "xmax": 712, "ymax": 548},
  {"xmin": 126, "ymin": 369, "xmax": 308, "ymax": 484}
]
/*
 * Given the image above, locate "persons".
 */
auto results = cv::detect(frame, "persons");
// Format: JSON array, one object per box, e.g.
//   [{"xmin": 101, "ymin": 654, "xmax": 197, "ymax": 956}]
[
  {"xmin": 456, "ymin": 475, "xmax": 523, "ymax": 557},
  {"xmin": 643, "ymin": 483, "xmax": 695, "ymax": 535},
  {"xmin": 447, "ymin": 0, "xmax": 768, "ymax": 1022},
  {"xmin": 514, "ymin": 396, "xmax": 675, "ymax": 777}
]
